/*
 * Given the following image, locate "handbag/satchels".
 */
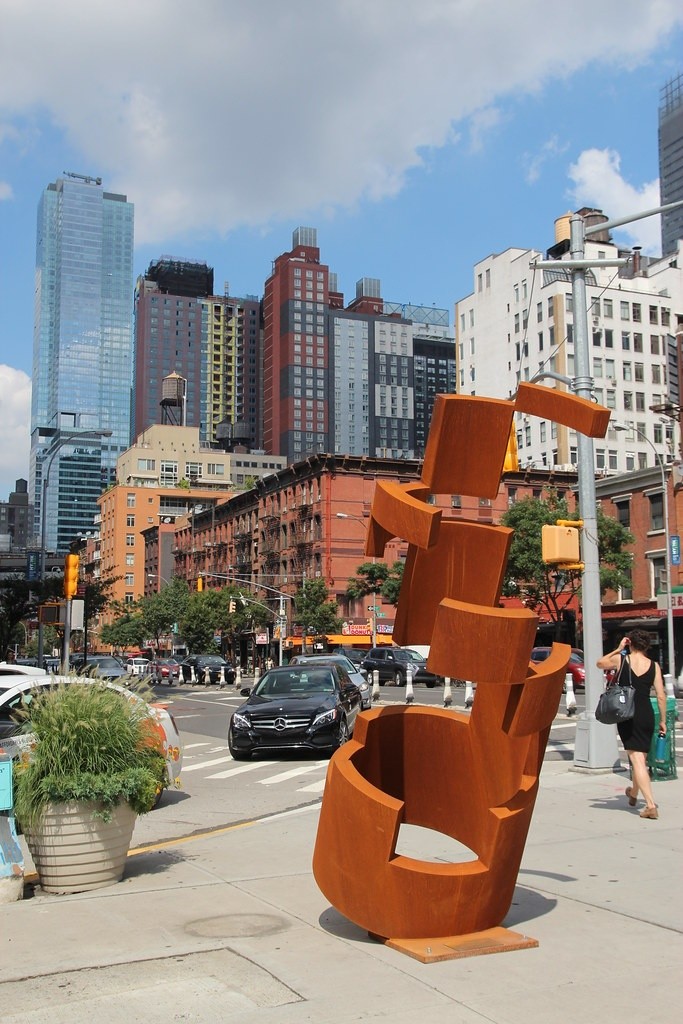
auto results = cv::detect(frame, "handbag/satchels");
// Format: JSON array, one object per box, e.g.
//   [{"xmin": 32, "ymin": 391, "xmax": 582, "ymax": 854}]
[{"xmin": 595, "ymin": 655, "xmax": 636, "ymax": 724}]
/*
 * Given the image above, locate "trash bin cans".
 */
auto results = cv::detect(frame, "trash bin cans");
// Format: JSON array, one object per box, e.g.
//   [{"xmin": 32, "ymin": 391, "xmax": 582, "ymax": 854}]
[{"xmin": 628, "ymin": 698, "xmax": 677, "ymax": 780}]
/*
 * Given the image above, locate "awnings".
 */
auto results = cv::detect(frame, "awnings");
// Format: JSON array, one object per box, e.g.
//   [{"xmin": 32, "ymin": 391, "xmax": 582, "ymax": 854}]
[{"xmin": 622, "ymin": 618, "xmax": 667, "ymax": 626}]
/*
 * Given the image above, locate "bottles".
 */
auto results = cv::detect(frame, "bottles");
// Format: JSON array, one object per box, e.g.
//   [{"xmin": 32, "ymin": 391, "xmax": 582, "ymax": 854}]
[{"xmin": 655, "ymin": 733, "xmax": 666, "ymax": 762}]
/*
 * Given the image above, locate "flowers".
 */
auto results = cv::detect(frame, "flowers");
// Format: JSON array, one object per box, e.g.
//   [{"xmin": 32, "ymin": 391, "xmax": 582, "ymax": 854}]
[{"xmin": 2, "ymin": 664, "xmax": 182, "ymax": 841}]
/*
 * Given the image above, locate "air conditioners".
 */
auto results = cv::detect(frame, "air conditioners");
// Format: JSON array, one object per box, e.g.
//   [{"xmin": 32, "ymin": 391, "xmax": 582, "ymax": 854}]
[
  {"xmin": 524, "ymin": 417, "xmax": 529, "ymax": 423},
  {"xmin": 593, "ymin": 316, "xmax": 599, "ymax": 322}
]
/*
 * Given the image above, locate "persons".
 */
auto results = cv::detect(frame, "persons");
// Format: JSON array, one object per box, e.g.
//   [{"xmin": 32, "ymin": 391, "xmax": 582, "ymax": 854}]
[
  {"xmin": 265, "ymin": 657, "xmax": 274, "ymax": 670},
  {"xmin": 2, "ymin": 651, "xmax": 18, "ymax": 665},
  {"xmin": 282, "ymin": 654, "xmax": 288, "ymax": 665},
  {"xmin": 597, "ymin": 631, "xmax": 667, "ymax": 818}
]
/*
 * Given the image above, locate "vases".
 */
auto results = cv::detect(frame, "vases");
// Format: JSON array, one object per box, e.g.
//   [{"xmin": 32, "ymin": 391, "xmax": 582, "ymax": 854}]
[{"xmin": 19, "ymin": 799, "xmax": 136, "ymax": 894}]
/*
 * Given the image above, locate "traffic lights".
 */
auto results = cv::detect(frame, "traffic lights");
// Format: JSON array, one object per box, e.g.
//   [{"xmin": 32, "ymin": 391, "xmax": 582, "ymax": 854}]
[
  {"xmin": 39, "ymin": 605, "xmax": 57, "ymax": 623},
  {"xmin": 229, "ymin": 602, "xmax": 236, "ymax": 613}
]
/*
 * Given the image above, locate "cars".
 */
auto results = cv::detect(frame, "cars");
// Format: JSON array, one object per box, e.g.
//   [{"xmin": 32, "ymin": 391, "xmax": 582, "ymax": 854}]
[
  {"xmin": 0, "ymin": 674, "xmax": 182, "ymax": 813},
  {"xmin": 359, "ymin": 646, "xmax": 437, "ymax": 688},
  {"xmin": 228, "ymin": 664, "xmax": 364, "ymax": 761},
  {"xmin": 123, "ymin": 658, "xmax": 149, "ymax": 677},
  {"xmin": 181, "ymin": 654, "xmax": 236, "ymax": 685},
  {"xmin": 147, "ymin": 658, "xmax": 180, "ymax": 677},
  {"xmin": 290, "ymin": 653, "xmax": 372, "ymax": 711},
  {"xmin": 15, "ymin": 653, "xmax": 132, "ymax": 691},
  {"xmin": 530, "ymin": 646, "xmax": 615, "ymax": 694},
  {"xmin": 0, "ymin": 662, "xmax": 48, "ymax": 675},
  {"xmin": 333, "ymin": 647, "xmax": 371, "ymax": 671}
]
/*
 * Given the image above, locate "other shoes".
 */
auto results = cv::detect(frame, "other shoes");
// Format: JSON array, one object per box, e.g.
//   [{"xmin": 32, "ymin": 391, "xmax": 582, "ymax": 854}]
[
  {"xmin": 626, "ymin": 786, "xmax": 637, "ymax": 806},
  {"xmin": 640, "ymin": 806, "xmax": 658, "ymax": 819}
]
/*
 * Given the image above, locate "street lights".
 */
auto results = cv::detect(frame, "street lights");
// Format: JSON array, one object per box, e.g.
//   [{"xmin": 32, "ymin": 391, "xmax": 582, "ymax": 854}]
[
  {"xmin": 36, "ymin": 429, "xmax": 112, "ymax": 670},
  {"xmin": 148, "ymin": 573, "xmax": 175, "ymax": 655},
  {"xmin": 615, "ymin": 422, "xmax": 676, "ymax": 679},
  {"xmin": 336, "ymin": 513, "xmax": 376, "ymax": 647}
]
